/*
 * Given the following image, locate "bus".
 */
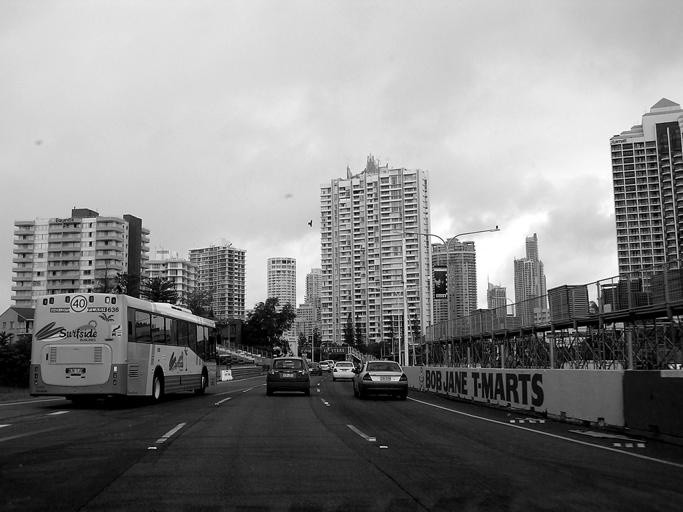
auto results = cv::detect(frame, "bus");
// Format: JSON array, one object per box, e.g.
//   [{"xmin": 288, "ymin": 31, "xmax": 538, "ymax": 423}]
[{"xmin": 29, "ymin": 292, "xmax": 217, "ymax": 405}]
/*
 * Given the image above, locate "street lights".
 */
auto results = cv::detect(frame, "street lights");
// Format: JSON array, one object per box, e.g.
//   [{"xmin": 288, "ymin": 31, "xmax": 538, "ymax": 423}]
[{"xmin": 392, "ymin": 225, "xmax": 502, "ymax": 367}]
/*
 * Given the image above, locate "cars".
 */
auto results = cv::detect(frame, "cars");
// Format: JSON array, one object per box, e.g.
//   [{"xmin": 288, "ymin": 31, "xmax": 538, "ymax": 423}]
[
  {"xmin": 306, "ymin": 359, "xmax": 355, "ymax": 381},
  {"xmin": 352, "ymin": 360, "xmax": 409, "ymax": 401},
  {"xmin": 265, "ymin": 357, "xmax": 310, "ymax": 396}
]
